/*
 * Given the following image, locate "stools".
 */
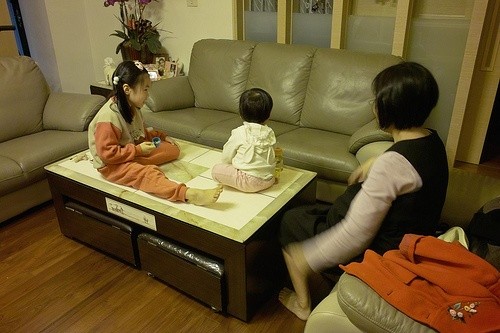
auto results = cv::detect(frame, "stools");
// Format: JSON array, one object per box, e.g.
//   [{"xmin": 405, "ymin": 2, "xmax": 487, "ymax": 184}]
[
  {"xmin": 63, "ymin": 201, "xmax": 140, "ymax": 266},
  {"xmin": 136, "ymin": 232, "xmax": 227, "ymax": 313}
]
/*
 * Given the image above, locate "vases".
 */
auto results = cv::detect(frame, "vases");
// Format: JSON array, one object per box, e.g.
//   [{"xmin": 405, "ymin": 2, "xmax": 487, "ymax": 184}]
[{"xmin": 120, "ymin": 46, "xmax": 155, "ymax": 64}]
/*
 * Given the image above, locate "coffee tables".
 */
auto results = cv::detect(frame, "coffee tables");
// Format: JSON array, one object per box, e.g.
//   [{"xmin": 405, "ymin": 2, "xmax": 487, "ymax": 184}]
[{"xmin": 43, "ymin": 135, "xmax": 317, "ymax": 324}]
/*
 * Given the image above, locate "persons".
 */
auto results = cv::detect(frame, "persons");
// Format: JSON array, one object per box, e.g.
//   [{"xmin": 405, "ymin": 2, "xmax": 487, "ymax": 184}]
[
  {"xmin": 88, "ymin": 61, "xmax": 223, "ymax": 205},
  {"xmin": 212, "ymin": 87, "xmax": 277, "ymax": 192},
  {"xmin": 278, "ymin": 61, "xmax": 449, "ymax": 320},
  {"xmin": 158, "ymin": 58, "xmax": 176, "ymax": 76}
]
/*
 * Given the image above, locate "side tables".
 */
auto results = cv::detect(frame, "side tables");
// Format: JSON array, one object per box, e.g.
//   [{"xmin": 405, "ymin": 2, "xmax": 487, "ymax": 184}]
[{"xmin": 90, "ymin": 80, "xmax": 113, "ymax": 99}]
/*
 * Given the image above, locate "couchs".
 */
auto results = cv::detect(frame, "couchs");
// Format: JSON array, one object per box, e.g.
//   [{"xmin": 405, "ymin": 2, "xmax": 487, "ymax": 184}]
[
  {"xmin": 0, "ymin": 56, "xmax": 106, "ymax": 223},
  {"xmin": 303, "ymin": 168, "xmax": 500, "ymax": 333},
  {"xmin": 141, "ymin": 39, "xmax": 403, "ymax": 205}
]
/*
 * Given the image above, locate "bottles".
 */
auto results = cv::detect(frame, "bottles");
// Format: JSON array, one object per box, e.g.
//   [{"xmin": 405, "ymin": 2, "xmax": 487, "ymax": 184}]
[
  {"xmin": 275, "ymin": 142, "xmax": 283, "ymax": 172},
  {"xmin": 103, "ymin": 57, "xmax": 117, "ymax": 86}
]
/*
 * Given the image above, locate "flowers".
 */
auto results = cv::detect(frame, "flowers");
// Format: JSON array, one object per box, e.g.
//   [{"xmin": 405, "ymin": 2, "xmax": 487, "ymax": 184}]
[{"xmin": 104, "ymin": 0, "xmax": 168, "ymax": 54}]
